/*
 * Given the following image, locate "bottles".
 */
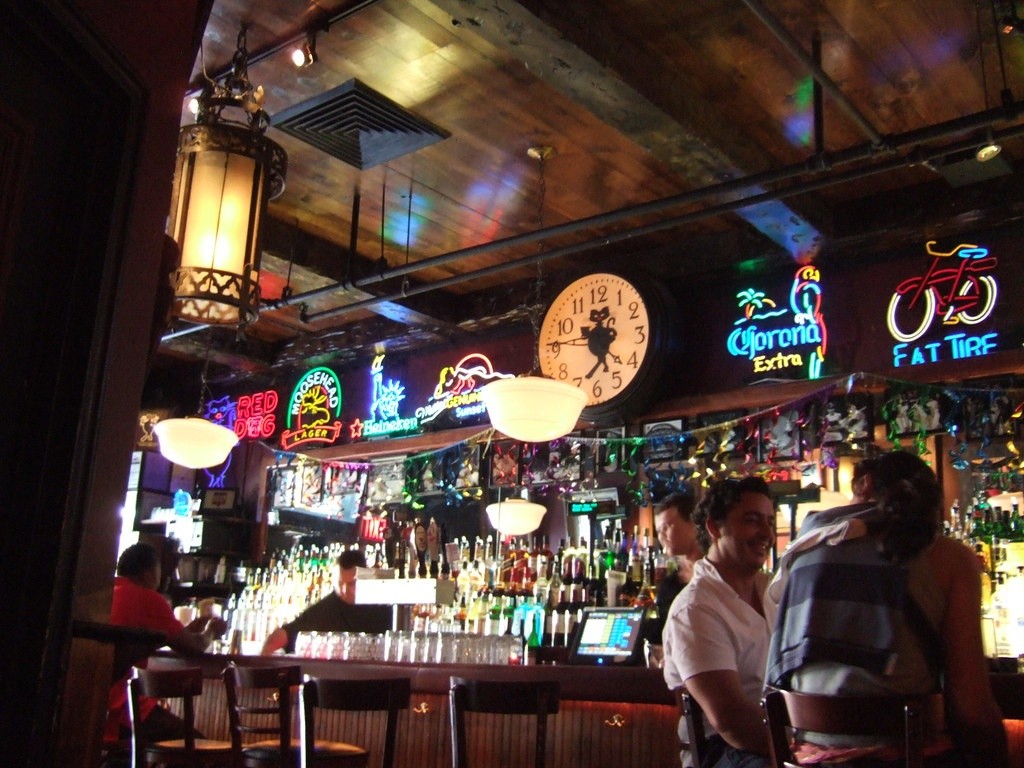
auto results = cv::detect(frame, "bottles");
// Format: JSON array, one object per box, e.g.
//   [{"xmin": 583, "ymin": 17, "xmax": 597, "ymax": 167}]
[
  {"xmin": 942, "ymin": 496, "xmax": 1024, "ymax": 657},
  {"xmin": 173, "ymin": 516, "xmax": 668, "ymax": 667}
]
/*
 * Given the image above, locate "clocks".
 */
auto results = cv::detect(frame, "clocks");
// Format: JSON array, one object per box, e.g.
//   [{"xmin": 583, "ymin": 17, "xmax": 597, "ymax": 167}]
[{"xmin": 536, "ymin": 271, "xmax": 658, "ymax": 422}]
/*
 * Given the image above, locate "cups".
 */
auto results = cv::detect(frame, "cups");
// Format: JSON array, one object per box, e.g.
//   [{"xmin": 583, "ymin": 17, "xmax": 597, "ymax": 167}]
[
  {"xmin": 213, "ymin": 640, "xmax": 231, "ymax": 654},
  {"xmin": 293, "ymin": 627, "xmax": 524, "ymax": 666}
]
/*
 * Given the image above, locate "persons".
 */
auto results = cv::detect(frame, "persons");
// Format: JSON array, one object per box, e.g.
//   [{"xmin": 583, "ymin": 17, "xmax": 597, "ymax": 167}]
[
  {"xmin": 261, "ymin": 550, "xmax": 393, "ymax": 656},
  {"xmin": 103, "ymin": 543, "xmax": 227, "ymax": 742},
  {"xmin": 762, "ymin": 451, "xmax": 1008, "ymax": 768},
  {"xmin": 423, "ymin": 462, "xmax": 433, "ymax": 491},
  {"xmin": 662, "ymin": 476, "xmax": 778, "ymax": 768},
  {"xmin": 654, "ymin": 492, "xmax": 705, "ymax": 623}
]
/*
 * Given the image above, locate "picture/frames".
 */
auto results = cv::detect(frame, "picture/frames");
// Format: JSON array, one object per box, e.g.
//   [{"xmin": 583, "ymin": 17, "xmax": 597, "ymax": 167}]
[
  {"xmin": 365, "ymin": 454, "xmax": 408, "ymax": 506},
  {"xmin": 328, "ymin": 458, "xmax": 362, "ymax": 495},
  {"xmin": 409, "ymin": 451, "xmax": 443, "ymax": 498},
  {"xmin": 756, "ymin": 404, "xmax": 805, "ymax": 464},
  {"xmin": 269, "ymin": 465, "xmax": 296, "ymax": 513},
  {"xmin": 694, "ymin": 407, "xmax": 750, "ymax": 462},
  {"xmin": 299, "ymin": 463, "xmax": 327, "ymax": 507},
  {"xmin": 445, "ymin": 445, "xmax": 482, "ymax": 494},
  {"xmin": 529, "ymin": 428, "xmax": 586, "ymax": 484},
  {"xmin": 885, "ymin": 387, "xmax": 951, "ymax": 442},
  {"xmin": 594, "ymin": 422, "xmax": 629, "ymax": 479},
  {"xmin": 199, "ymin": 487, "xmax": 238, "ymax": 516},
  {"xmin": 635, "ymin": 416, "xmax": 691, "ymax": 465},
  {"xmin": 488, "ymin": 437, "xmax": 523, "ymax": 490},
  {"xmin": 820, "ymin": 393, "xmax": 875, "ymax": 447}
]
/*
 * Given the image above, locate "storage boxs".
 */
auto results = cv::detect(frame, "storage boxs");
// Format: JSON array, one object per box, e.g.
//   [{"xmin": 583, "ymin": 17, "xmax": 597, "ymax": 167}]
[{"xmin": 355, "ymin": 578, "xmax": 455, "ymax": 606}]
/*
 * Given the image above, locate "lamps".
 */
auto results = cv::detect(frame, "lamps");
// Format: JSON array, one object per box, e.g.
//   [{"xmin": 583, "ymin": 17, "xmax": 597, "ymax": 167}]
[
  {"xmin": 152, "ymin": 327, "xmax": 241, "ymax": 469},
  {"xmin": 286, "ymin": 19, "xmax": 330, "ymax": 66},
  {"xmin": 485, "ymin": 442, "xmax": 547, "ymax": 535},
  {"xmin": 161, "ymin": 24, "xmax": 275, "ymax": 332},
  {"xmin": 479, "ymin": 143, "xmax": 591, "ymax": 443}
]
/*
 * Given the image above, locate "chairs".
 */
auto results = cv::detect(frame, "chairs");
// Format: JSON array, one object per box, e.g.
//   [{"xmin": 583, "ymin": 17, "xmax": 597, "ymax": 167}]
[
  {"xmin": 298, "ymin": 673, "xmax": 412, "ymax": 768},
  {"xmin": 673, "ymin": 690, "xmax": 711, "ymax": 768},
  {"xmin": 760, "ymin": 685, "xmax": 946, "ymax": 768},
  {"xmin": 125, "ymin": 663, "xmax": 232, "ymax": 768},
  {"xmin": 221, "ymin": 661, "xmax": 368, "ymax": 768},
  {"xmin": 448, "ymin": 675, "xmax": 558, "ymax": 768}
]
